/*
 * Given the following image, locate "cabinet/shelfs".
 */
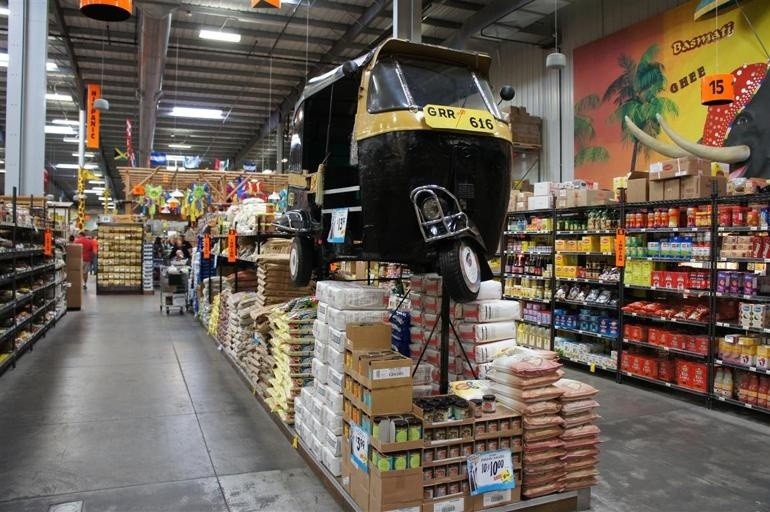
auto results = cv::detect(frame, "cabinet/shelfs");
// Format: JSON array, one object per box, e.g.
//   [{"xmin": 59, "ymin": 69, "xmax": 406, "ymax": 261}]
[
  {"xmin": 513, "ymin": 178, "xmax": 769, "ymax": 418},
  {"xmin": 0, "ymin": 188, "xmax": 69, "ymax": 373},
  {"xmin": 96, "ymin": 215, "xmax": 154, "ymax": 295},
  {"xmin": 189, "ymin": 199, "xmax": 602, "ymax": 512}
]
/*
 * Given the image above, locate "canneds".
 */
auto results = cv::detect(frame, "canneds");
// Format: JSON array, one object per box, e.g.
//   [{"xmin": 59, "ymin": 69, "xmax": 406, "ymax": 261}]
[{"xmin": 372, "ymin": 393, "xmax": 521, "ymax": 499}]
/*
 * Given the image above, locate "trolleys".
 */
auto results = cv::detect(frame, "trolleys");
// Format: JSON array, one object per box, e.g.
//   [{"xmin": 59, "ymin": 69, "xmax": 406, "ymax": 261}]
[{"xmin": 159, "ymin": 256, "xmax": 191, "ymax": 315}]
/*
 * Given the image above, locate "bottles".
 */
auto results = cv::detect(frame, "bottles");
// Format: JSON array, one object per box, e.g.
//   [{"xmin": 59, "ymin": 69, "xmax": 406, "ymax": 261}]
[
  {"xmin": 712, "ymin": 367, "xmax": 769, "ymax": 408},
  {"xmin": 624, "ymin": 205, "xmax": 699, "ymax": 228},
  {"xmin": 508, "ymin": 214, "xmax": 529, "ymax": 233},
  {"xmin": 555, "ymin": 211, "xmax": 586, "ymax": 231},
  {"xmin": 503, "ymin": 254, "xmax": 552, "ymax": 279}
]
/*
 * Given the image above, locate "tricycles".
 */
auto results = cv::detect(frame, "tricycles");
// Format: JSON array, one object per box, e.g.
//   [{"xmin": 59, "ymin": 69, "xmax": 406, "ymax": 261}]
[{"xmin": 273, "ymin": 40, "xmax": 517, "ymax": 304}]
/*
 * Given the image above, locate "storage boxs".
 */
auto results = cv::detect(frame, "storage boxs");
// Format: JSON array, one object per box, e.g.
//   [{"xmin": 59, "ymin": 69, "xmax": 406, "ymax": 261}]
[
  {"xmin": 66, "ymin": 243, "xmax": 83, "ymax": 311},
  {"xmin": 509, "ymin": 156, "xmax": 731, "ymax": 212},
  {"xmin": 503, "ymin": 106, "xmax": 542, "ymax": 149}
]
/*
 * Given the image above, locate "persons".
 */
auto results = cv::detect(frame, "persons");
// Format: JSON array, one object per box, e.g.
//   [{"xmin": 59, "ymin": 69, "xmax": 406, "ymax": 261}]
[
  {"xmin": 69, "ymin": 235, "xmax": 75, "ymax": 242},
  {"xmin": 169, "ymin": 234, "xmax": 192, "ymax": 259},
  {"xmin": 74, "ymin": 232, "xmax": 92, "ymax": 289},
  {"xmin": 152, "ymin": 237, "xmax": 163, "ymax": 287},
  {"xmin": 90, "ymin": 235, "xmax": 97, "ymax": 274}
]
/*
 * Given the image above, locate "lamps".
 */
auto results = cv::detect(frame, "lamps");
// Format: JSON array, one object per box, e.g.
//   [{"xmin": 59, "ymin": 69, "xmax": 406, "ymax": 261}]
[
  {"xmin": 94, "ymin": 19, "xmax": 109, "ymax": 113},
  {"xmin": 546, "ymin": 0, "xmax": 566, "ymax": 70},
  {"xmin": 701, "ymin": 0, "xmax": 735, "ymax": 106}
]
